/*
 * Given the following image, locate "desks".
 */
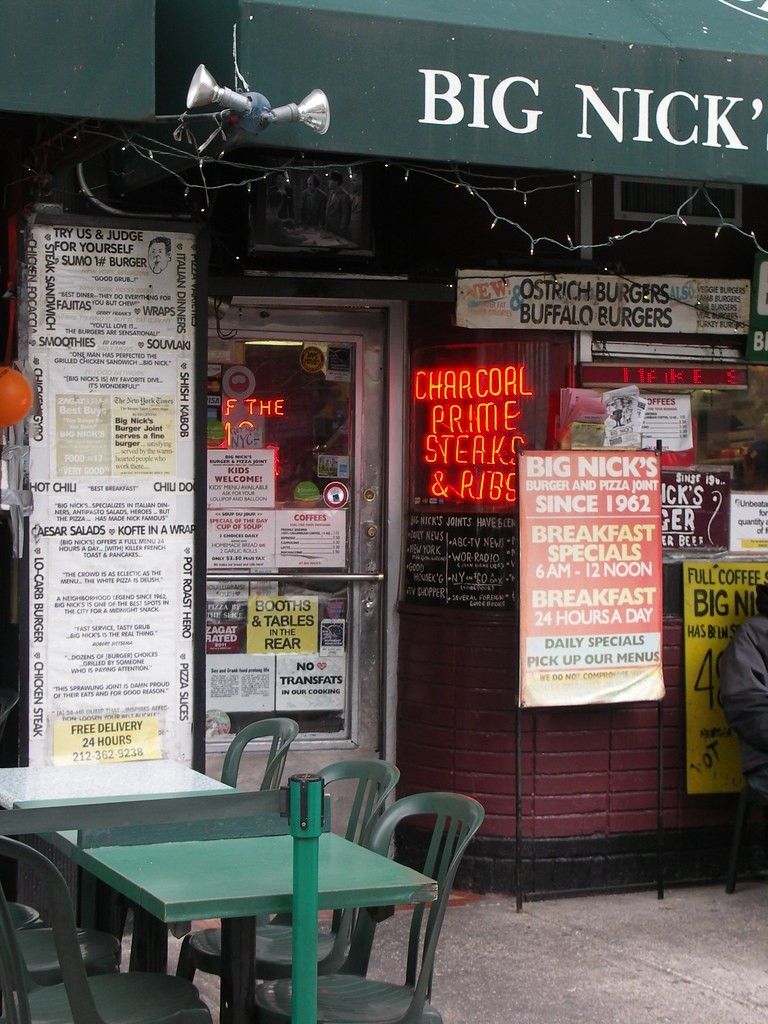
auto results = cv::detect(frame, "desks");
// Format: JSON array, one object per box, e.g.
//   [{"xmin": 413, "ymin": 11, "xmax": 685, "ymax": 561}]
[
  {"xmin": 80, "ymin": 831, "xmax": 439, "ymax": 924},
  {"xmin": 0, "ymin": 761, "xmax": 238, "ymax": 810}
]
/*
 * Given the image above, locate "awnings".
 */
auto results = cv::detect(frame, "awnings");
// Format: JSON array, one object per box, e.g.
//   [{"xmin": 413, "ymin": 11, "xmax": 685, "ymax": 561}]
[
  {"xmin": 1, "ymin": 0, "xmax": 156, "ymax": 173},
  {"xmin": 117, "ymin": 0, "xmax": 768, "ymax": 199}
]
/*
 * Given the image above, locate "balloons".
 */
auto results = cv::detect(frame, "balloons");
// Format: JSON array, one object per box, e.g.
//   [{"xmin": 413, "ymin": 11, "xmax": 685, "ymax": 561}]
[{"xmin": 0, "ymin": 366, "xmax": 33, "ymax": 428}]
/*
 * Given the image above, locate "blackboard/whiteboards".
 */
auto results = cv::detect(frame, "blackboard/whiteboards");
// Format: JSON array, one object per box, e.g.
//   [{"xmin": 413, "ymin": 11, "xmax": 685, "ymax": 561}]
[{"xmin": 405, "ymin": 511, "xmax": 517, "ymax": 610}]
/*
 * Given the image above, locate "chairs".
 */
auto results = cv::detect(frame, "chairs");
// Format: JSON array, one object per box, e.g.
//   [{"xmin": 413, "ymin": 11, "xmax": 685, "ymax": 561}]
[
  {"xmin": 175, "ymin": 757, "xmax": 400, "ymax": 981},
  {"xmin": 15, "ymin": 927, "xmax": 122, "ymax": 987},
  {"xmin": 253, "ymin": 790, "xmax": 486, "ymax": 1024},
  {"xmin": 0, "ymin": 837, "xmax": 214, "ymax": 1024},
  {"xmin": 725, "ymin": 775, "xmax": 768, "ymax": 895},
  {"xmin": 221, "ymin": 716, "xmax": 300, "ymax": 790}
]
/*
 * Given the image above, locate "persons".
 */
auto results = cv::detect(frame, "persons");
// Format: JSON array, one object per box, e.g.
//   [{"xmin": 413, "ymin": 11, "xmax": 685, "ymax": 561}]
[
  {"xmin": 264, "ymin": 170, "xmax": 363, "ymax": 245},
  {"xmin": 742, "ymin": 438, "xmax": 768, "ymax": 491},
  {"xmin": 718, "ymin": 581, "xmax": 768, "ymax": 796}
]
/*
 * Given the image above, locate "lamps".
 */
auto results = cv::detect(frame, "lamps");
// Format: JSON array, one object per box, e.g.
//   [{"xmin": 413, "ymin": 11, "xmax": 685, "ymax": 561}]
[
  {"xmin": 263, "ymin": 88, "xmax": 332, "ymax": 136},
  {"xmin": 185, "ymin": 63, "xmax": 253, "ymax": 112}
]
